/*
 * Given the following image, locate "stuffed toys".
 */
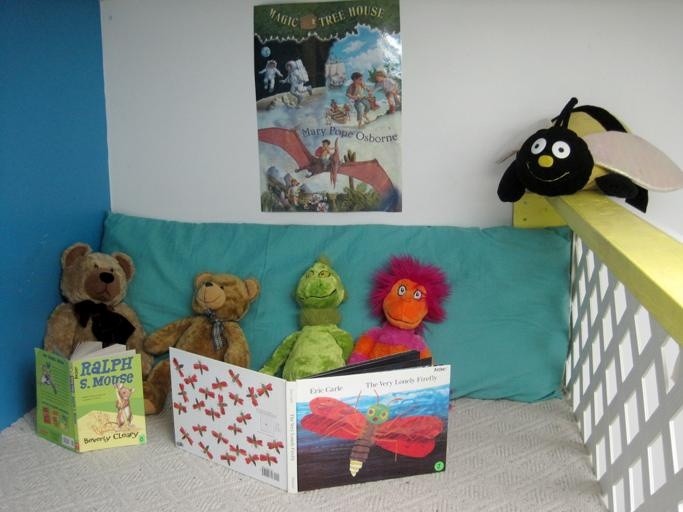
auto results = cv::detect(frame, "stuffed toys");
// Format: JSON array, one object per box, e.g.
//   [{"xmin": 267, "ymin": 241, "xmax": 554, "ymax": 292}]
[
  {"xmin": 41, "ymin": 240, "xmax": 156, "ymax": 378},
  {"xmin": 345, "ymin": 253, "xmax": 450, "ymax": 370},
  {"xmin": 256, "ymin": 255, "xmax": 357, "ymax": 379},
  {"xmin": 493, "ymin": 96, "xmax": 682, "ymax": 214},
  {"xmin": 140, "ymin": 272, "xmax": 260, "ymax": 415}
]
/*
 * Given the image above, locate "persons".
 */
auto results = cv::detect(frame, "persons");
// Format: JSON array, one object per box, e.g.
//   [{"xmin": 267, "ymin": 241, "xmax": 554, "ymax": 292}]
[
  {"xmin": 286, "ymin": 177, "xmax": 303, "ymax": 207},
  {"xmin": 276, "ymin": 57, "xmax": 312, "ymax": 103},
  {"xmin": 258, "ymin": 59, "xmax": 284, "ymax": 91},
  {"xmin": 374, "ymin": 70, "xmax": 400, "ymax": 114},
  {"xmin": 318, "ymin": 139, "xmax": 336, "ymax": 169},
  {"xmin": 346, "ymin": 71, "xmax": 371, "ymax": 128}
]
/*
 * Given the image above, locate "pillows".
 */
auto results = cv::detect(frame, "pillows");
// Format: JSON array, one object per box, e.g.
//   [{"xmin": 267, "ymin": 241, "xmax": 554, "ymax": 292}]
[{"xmin": 96, "ymin": 210, "xmax": 574, "ymax": 411}]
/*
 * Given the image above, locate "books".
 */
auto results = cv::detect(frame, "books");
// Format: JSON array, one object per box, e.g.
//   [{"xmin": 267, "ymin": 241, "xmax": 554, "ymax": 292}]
[
  {"xmin": 164, "ymin": 338, "xmax": 450, "ymax": 494},
  {"xmin": 31, "ymin": 338, "xmax": 150, "ymax": 455}
]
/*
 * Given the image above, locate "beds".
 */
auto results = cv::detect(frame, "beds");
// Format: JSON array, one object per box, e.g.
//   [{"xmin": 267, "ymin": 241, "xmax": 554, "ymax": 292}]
[{"xmin": 1, "ymin": 179, "xmax": 683, "ymax": 511}]
